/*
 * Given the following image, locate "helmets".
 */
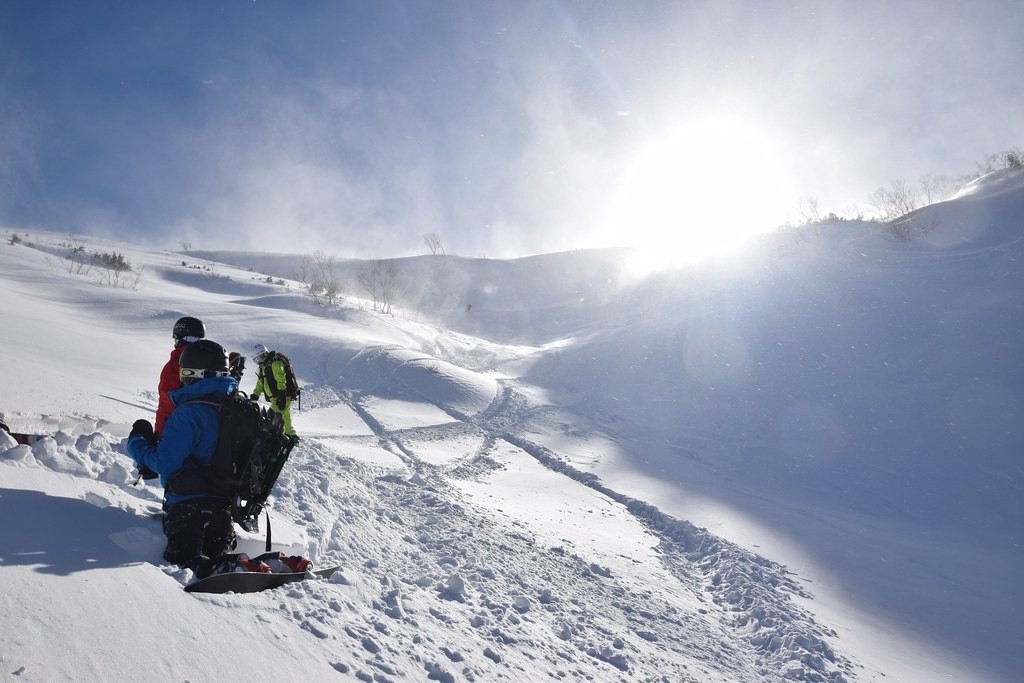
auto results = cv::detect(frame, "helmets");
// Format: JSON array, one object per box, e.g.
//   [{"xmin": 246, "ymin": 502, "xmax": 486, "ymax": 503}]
[
  {"xmin": 249, "ymin": 344, "xmax": 269, "ymax": 363},
  {"xmin": 179, "ymin": 340, "xmax": 228, "ymax": 381},
  {"xmin": 173, "ymin": 317, "xmax": 205, "ymax": 338}
]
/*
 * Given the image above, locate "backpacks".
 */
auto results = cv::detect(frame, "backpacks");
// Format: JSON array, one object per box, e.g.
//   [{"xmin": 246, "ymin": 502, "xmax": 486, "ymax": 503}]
[
  {"xmin": 165, "ymin": 390, "xmax": 298, "ymax": 552},
  {"xmin": 260, "ymin": 350, "xmax": 301, "ymax": 411}
]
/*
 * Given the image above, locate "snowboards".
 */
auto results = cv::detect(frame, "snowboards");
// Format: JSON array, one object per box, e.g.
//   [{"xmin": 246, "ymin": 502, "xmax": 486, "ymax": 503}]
[{"xmin": 183, "ymin": 566, "xmax": 342, "ymax": 593}]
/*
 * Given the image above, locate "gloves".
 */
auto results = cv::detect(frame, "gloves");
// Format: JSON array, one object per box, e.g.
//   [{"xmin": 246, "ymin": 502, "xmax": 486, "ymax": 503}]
[
  {"xmin": 251, "ymin": 394, "xmax": 258, "ymax": 400},
  {"xmin": 276, "ymin": 392, "xmax": 286, "ymax": 410},
  {"xmin": 130, "ymin": 419, "xmax": 159, "ymax": 480}
]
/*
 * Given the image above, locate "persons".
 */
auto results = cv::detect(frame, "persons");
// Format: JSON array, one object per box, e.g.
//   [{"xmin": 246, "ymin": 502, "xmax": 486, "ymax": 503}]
[
  {"xmin": 129, "ymin": 341, "xmax": 282, "ymax": 579},
  {"xmin": 248, "ymin": 342, "xmax": 301, "ymax": 445},
  {"xmin": 138, "ymin": 316, "xmax": 207, "ymax": 480}
]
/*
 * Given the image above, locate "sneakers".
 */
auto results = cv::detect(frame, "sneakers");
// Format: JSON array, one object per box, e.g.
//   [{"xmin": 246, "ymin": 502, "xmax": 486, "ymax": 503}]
[
  {"xmin": 237, "ymin": 559, "xmax": 270, "ymax": 573},
  {"xmin": 281, "ymin": 556, "xmax": 312, "ymax": 572}
]
